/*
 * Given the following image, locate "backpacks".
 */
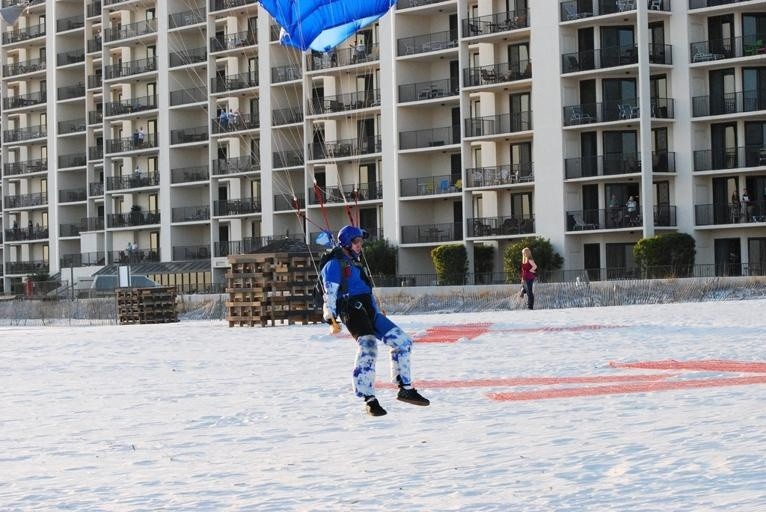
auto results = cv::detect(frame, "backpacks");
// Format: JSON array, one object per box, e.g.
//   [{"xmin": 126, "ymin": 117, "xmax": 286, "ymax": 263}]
[{"xmin": 313, "ymin": 246, "xmax": 351, "ymax": 307}]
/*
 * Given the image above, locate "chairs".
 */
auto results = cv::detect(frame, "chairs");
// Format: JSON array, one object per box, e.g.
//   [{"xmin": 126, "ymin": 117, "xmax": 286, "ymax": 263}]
[
  {"xmin": 573, "ymin": 214, "xmax": 597, "ymax": 230},
  {"xmin": 563, "ymin": 104, "xmax": 595, "ymax": 125},
  {"xmin": 568, "ymin": 56, "xmax": 594, "ymax": 71},
  {"xmin": 617, "ymin": 103, "xmax": 639, "ymax": 119}
]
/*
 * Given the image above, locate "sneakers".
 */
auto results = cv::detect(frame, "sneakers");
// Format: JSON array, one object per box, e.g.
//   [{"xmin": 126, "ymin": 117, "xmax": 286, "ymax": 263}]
[
  {"xmin": 366, "ymin": 399, "xmax": 386, "ymax": 416},
  {"xmin": 397, "ymin": 387, "xmax": 429, "ymax": 406}
]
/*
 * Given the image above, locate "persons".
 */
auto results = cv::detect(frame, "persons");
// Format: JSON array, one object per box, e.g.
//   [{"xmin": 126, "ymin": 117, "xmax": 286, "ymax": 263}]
[
  {"xmin": 350, "ymin": 40, "xmax": 358, "ymax": 64},
  {"xmin": 219, "ymin": 108, "xmax": 228, "ymax": 132},
  {"xmin": 132, "ymin": 128, "xmax": 138, "ymax": 149},
  {"xmin": 329, "ymin": 48, "xmax": 340, "ymax": 68},
  {"xmin": 624, "ymin": 195, "xmax": 637, "ymax": 226},
  {"xmin": 139, "ymin": 125, "xmax": 146, "ymax": 149},
  {"xmin": 356, "ymin": 39, "xmax": 366, "ymax": 62},
  {"xmin": 125, "ymin": 241, "xmax": 132, "ymax": 263},
  {"xmin": 316, "ymin": 225, "xmax": 433, "ymax": 419},
  {"xmin": 228, "ymin": 108, "xmax": 238, "ymax": 132},
  {"xmin": 518, "ymin": 246, "xmax": 538, "ymax": 311},
  {"xmin": 131, "ymin": 240, "xmax": 141, "ymax": 265},
  {"xmin": 730, "ymin": 189, "xmax": 740, "ymax": 224},
  {"xmin": 133, "ymin": 164, "xmax": 142, "ymax": 187},
  {"xmin": 608, "ymin": 193, "xmax": 621, "ymax": 228},
  {"xmin": 741, "ymin": 187, "xmax": 751, "ymax": 223}
]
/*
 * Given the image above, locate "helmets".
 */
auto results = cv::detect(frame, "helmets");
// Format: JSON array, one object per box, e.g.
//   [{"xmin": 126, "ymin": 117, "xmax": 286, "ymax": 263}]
[{"xmin": 338, "ymin": 226, "xmax": 363, "ymax": 248}]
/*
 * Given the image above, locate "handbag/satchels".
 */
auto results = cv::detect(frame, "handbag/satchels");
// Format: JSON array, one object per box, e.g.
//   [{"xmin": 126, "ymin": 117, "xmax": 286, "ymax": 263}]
[{"xmin": 521, "ymin": 286, "xmax": 526, "ymax": 293}]
